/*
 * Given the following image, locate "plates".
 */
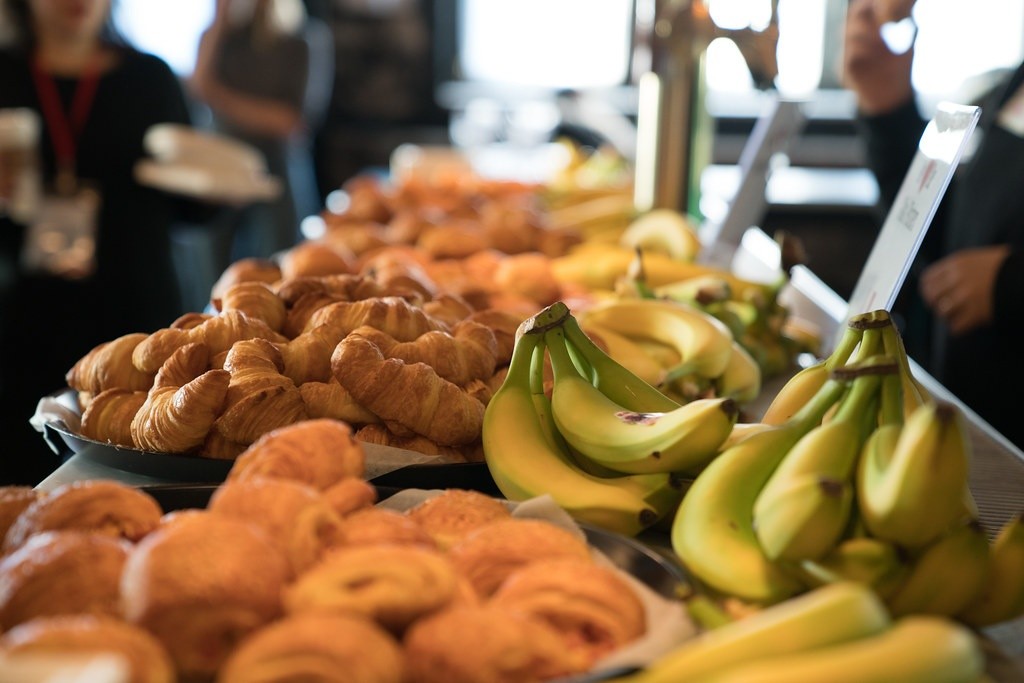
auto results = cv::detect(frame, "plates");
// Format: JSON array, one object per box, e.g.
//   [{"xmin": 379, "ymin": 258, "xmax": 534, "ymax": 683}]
[
  {"xmin": 133, "ymin": 485, "xmax": 219, "ymax": 515},
  {"xmin": 358, "ymin": 458, "xmax": 500, "ymax": 502},
  {"xmin": 41, "ymin": 391, "xmax": 237, "ymax": 483}
]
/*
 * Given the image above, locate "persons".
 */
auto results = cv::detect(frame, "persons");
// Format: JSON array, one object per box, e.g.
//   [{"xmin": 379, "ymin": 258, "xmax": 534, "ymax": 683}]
[
  {"xmin": 176, "ymin": 1, "xmax": 316, "ymax": 293},
  {"xmin": 0, "ymin": 0, "xmax": 224, "ymax": 518},
  {"xmin": 839, "ymin": 1, "xmax": 1024, "ymax": 443}
]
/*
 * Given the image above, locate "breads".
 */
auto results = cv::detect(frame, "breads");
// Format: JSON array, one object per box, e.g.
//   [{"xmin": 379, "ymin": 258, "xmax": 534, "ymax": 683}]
[
  {"xmin": 1, "ymin": 419, "xmax": 648, "ymax": 683},
  {"xmin": 65, "ymin": 275, "xmax": 528, "ymax": 462}
]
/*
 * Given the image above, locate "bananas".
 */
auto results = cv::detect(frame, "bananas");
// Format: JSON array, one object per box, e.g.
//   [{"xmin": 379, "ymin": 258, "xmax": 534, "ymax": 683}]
[
  {"xmin": 482, "ymin": 246, "xmax": 824, "ymax": 537},
  {"xmin": 608, "ymin": 308, "xmax": 1024, "ymax": 683}
]
